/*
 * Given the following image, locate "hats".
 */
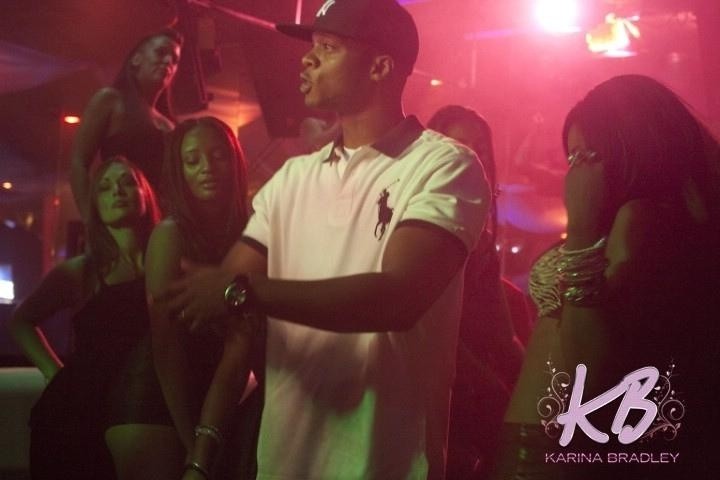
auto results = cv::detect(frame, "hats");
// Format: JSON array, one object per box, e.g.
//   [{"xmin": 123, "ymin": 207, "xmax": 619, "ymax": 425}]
[{"xmin": 275, "ymin": 0, "xmax": 419, "ymax": 66}]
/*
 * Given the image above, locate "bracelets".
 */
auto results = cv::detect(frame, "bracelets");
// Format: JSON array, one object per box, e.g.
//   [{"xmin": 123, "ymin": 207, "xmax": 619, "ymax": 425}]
[
  {"xmin": 558, "ymin": 236, "xmax": 613, "ymax": 306},
  {"xmin": 191, "ymin": 423, "xmax": 227, "ymax": 448},
  {"xmin": 183, "ymin": 459, "xmax": 211, "ymax": 478}
]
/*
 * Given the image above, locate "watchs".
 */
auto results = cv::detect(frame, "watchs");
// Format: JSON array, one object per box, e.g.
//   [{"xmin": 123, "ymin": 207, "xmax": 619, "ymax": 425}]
[{"xmin": 220, "ymin": 271, "xmax": 250, "ymax": 318}]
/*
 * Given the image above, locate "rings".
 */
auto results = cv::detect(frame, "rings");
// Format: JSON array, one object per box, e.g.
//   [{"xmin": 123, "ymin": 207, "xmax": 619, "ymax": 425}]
[
  {"xmin": 180, "ymin": 309, "xmax": 186, "ymax": 324},
  {"xmin": 586, "ymin": 152, "xmax": 598, "ymax": 162},
  {"xmin": 566, "ymin": 154, "xmax": 576, "ymax": 164}
]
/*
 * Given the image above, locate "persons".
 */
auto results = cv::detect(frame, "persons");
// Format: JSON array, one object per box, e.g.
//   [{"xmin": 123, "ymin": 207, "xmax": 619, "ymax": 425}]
[
  {"xmin": 70, "ymin": 26, "xmax": 185, "ymax": 246},
  {"xmin": 496, "ymin": 72, "xmax": 720, "ymax": 478},
  {"xmin": 419, "ymin": 98, "xmax": 536, "ymax": 479},
  {"xmin": 106, "ymin": 114, "xmax": 253, "ymax": 478},
  {"xmin": 5, "ymin": 155, "xmax": 164, "ymax": 480},
  {"xmin": 147, "ymin": 0, "xmax": 496, "ymax": 480}
]
